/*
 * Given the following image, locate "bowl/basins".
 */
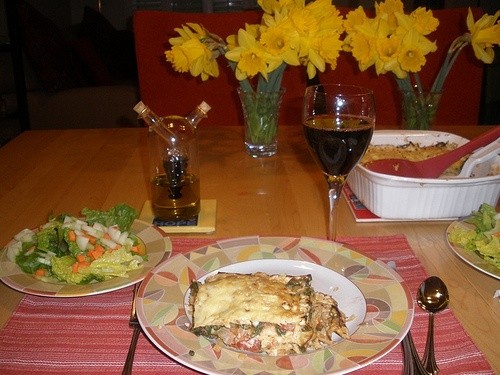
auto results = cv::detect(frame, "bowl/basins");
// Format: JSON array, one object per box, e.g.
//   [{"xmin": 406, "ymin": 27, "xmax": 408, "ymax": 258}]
[{"xmin": 346, "ymin": 130, "xmax": 500, "ymax": 219}]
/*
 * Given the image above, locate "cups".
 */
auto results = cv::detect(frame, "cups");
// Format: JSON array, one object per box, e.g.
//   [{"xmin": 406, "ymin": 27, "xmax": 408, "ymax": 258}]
[
  {"xmin": 237, "ymin": 86, "xmax": 286, "ymax": 157},
  {"xmin": 400, "ymin": 90, "xmax": 441, "ymax": 130}
]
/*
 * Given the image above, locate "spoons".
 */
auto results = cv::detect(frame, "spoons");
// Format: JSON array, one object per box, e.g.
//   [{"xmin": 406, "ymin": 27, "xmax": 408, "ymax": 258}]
[{"xmin": 416, "ymin": 276, "xmax": 449, "ymax": 375}]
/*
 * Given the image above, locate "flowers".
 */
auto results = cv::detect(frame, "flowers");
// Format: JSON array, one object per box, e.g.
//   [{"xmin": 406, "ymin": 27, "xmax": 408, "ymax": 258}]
[
  {"xmin": 165, "ymin": 0, "xmax": 343, "ymax": 89},
  {"xmin": 341, "ymin": 0, "xmax": 500, "ymax": 90}
]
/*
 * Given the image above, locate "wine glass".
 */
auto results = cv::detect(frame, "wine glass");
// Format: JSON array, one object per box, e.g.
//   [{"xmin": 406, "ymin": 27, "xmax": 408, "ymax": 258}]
[{"xmin": 302, "ymin": 83, "xmax": 376, "ymax": 241}]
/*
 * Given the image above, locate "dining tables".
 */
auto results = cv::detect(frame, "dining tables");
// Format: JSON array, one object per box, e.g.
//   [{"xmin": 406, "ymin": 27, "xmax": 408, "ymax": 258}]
[{"xmin": 0, "ymin": 126, "xmax": 500, "ymax": 375}]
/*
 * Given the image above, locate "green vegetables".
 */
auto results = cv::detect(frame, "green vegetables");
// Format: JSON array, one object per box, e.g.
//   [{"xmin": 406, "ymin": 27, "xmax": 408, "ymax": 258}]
[
  {"xmin": 449, "ymin": 202, "xmax": 500, "ymax": 269},
  {"xmin": 7, "ymin": 204, "xmax": 148, "ymax": 285}
]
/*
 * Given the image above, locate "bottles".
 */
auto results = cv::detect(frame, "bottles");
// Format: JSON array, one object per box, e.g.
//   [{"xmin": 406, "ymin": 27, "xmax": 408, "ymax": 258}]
[{"xmin": 134, "ymin": 101, "xmax": 211, "ymax": 221}]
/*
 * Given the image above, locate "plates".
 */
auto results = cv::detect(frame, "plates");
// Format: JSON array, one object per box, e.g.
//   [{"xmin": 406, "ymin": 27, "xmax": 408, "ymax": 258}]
[
  {"xmin": 135, "ymin": 234, "xmax": 415, "ymax": 375},
  {"xmin": 0, "ymin": 218, "xmax": 172, "ymax": 297},
  {"xmin": 445, "ymin": 217, "xmax": 500, "ymax": 280}
]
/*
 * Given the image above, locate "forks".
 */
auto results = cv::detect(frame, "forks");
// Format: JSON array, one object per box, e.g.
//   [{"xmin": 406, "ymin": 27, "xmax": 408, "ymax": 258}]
[{"xmin": 121, "ymin": 281, "xmax": 141, "ymax": 375}]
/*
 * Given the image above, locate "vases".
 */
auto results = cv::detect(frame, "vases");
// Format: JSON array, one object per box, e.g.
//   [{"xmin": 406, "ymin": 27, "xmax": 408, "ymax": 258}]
[
  {"xmin": 401, "ymin": 89, "xmax": 441, "ymax": 129},
  {"xmin": 236, "ymin": 88, "xmax": 285, "ymax": 159}
]
